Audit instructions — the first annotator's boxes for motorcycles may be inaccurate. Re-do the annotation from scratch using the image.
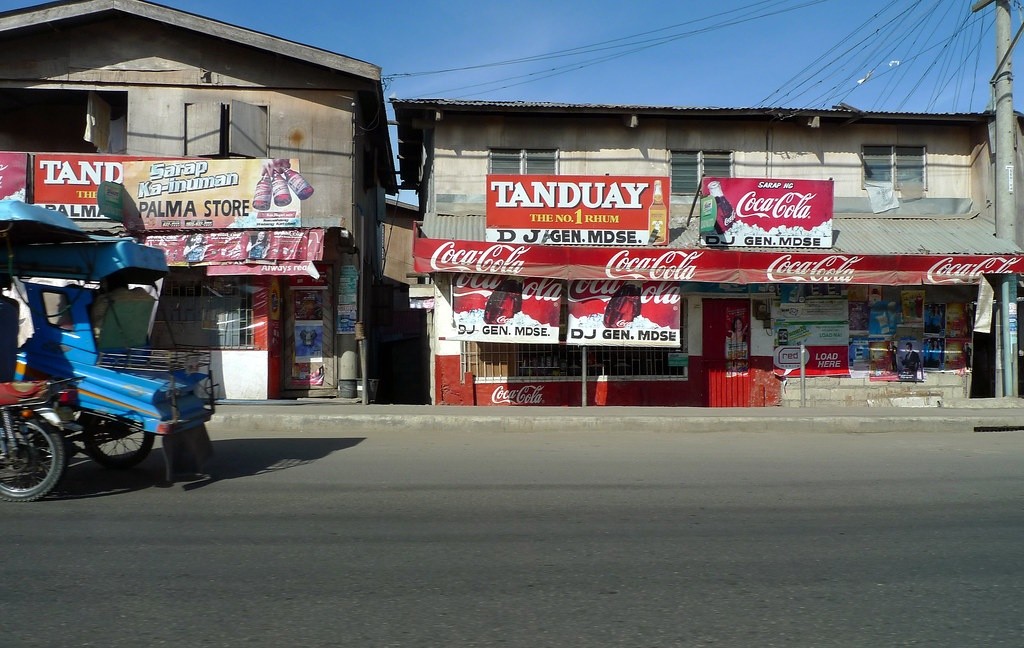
[0,376,87,504]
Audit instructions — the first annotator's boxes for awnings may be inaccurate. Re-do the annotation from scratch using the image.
[411,215,1023,285]
[87,225,359,278]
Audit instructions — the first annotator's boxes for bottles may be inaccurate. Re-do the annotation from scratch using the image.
[272,169,292,206]
[280,166,314,200]
[649,180,667,244]
[708,182,737,236]
[252,170,272,210]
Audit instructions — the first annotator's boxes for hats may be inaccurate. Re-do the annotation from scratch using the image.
[965,343,969,345]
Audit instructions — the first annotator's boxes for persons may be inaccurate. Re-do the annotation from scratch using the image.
[728,316,743,342]
[183,233,210,263]
[246,231,271,259]
[929,305,942,333]
[899,342,919,381]
[56,270,128,330]
[929,339,942,368]
